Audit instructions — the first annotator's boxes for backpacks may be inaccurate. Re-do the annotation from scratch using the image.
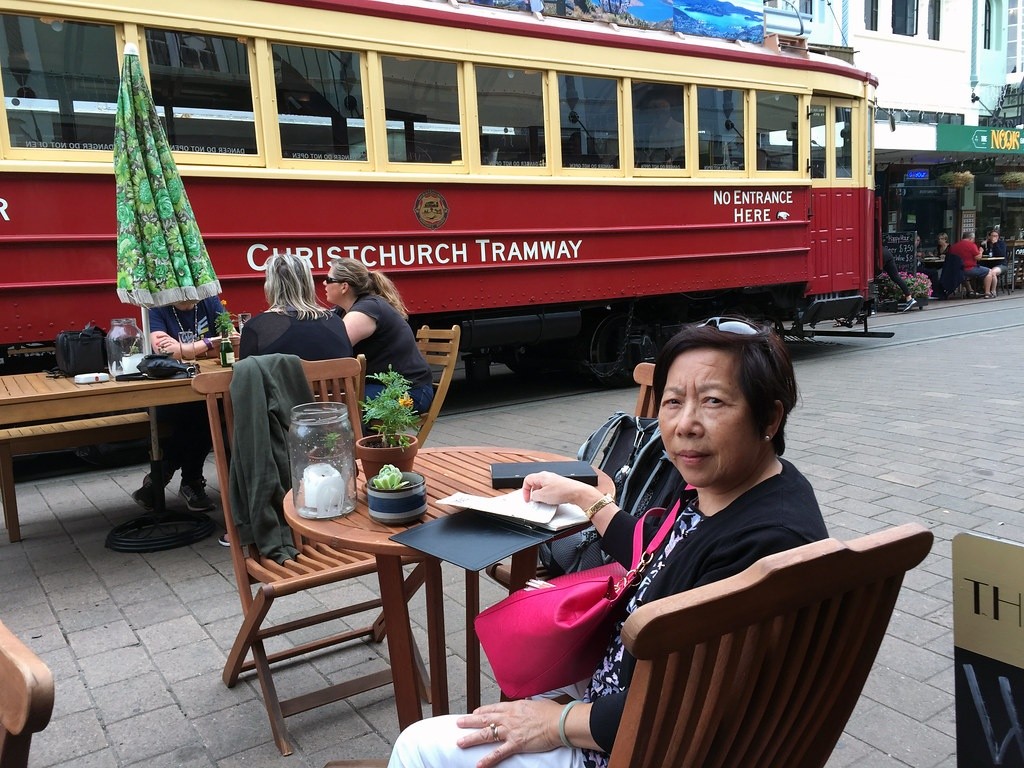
[538,410,689,578]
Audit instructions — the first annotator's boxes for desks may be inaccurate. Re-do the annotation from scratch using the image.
[0,353,240,426]
[283,445,615,717]
[924,257,1005,262]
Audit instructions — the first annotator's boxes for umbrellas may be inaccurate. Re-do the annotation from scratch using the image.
[114,43,223,362]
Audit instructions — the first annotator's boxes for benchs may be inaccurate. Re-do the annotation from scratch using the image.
[0,412,174,544]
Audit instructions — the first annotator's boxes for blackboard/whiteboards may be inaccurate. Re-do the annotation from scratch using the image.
[882,230,918,275]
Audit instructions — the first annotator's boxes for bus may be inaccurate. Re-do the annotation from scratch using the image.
[0,0,898,394]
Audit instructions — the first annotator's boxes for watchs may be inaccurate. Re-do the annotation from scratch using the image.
[585,492,616,519]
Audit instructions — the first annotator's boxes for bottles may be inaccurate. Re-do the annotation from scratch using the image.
[106,318,149,378]
[287,401,357,521]
[219,338,235,367]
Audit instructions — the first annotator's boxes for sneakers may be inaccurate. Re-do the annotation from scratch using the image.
[142,465,175,509]
[177,479,218,513]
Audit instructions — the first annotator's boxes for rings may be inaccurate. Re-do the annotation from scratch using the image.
[491,723,500,743]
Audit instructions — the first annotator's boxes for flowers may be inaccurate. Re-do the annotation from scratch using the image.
[359,364,423,449]
[941,171,975,190]
[214,300,234,338]
[877,272,933,296]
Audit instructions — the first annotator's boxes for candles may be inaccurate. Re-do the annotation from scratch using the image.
[120,353,145,374]
[303,462,344,509]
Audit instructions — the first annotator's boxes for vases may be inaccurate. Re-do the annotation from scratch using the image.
[210,336,240,365]
[355,433,418,496]
[951,176,964,188]
[914,294,929,307]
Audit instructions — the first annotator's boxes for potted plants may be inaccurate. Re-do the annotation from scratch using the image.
[367,464,428,524]
[1000,171,1024,190]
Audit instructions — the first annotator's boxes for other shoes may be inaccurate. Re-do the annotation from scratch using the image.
[903,298,917,313]
[967,290,998,299]
[217,534,231,548]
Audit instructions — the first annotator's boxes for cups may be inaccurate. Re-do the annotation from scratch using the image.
[238,313,251,335]
[178,332,196,364]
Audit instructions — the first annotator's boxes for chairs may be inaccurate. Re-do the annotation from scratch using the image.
[607,521,935,768]
[372,324,463,448]
[312,353,367,423]
[997,250,1012,295]
[191,357,432,756]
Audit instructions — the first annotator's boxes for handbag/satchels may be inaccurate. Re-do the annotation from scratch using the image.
[473,482,696,702]
[136,351,196,380]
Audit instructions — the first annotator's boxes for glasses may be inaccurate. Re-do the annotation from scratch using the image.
[989,234,999,237]
[938,237,945,241]
[680,314,762,334]
[324,275,343,285]
[917,239,921,243]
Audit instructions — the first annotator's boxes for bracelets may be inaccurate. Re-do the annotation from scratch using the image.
[559,700,584,749]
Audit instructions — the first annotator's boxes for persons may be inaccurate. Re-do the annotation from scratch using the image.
[915,229,1009,299]
[872,247,916,314]
[323,257,432,434]
[238,255,353,362]
[132,295,238,511]
[383,315,832,768]
